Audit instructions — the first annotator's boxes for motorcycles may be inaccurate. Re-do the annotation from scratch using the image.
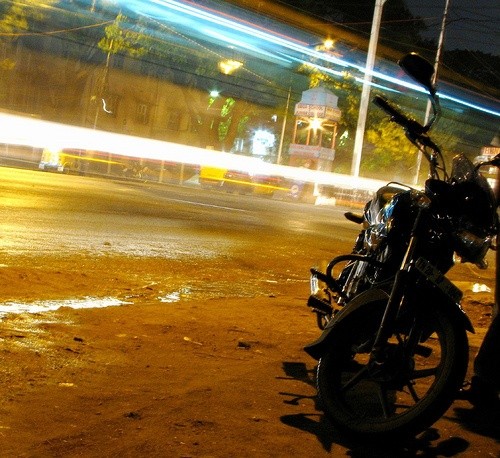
[306,51,500,447]
